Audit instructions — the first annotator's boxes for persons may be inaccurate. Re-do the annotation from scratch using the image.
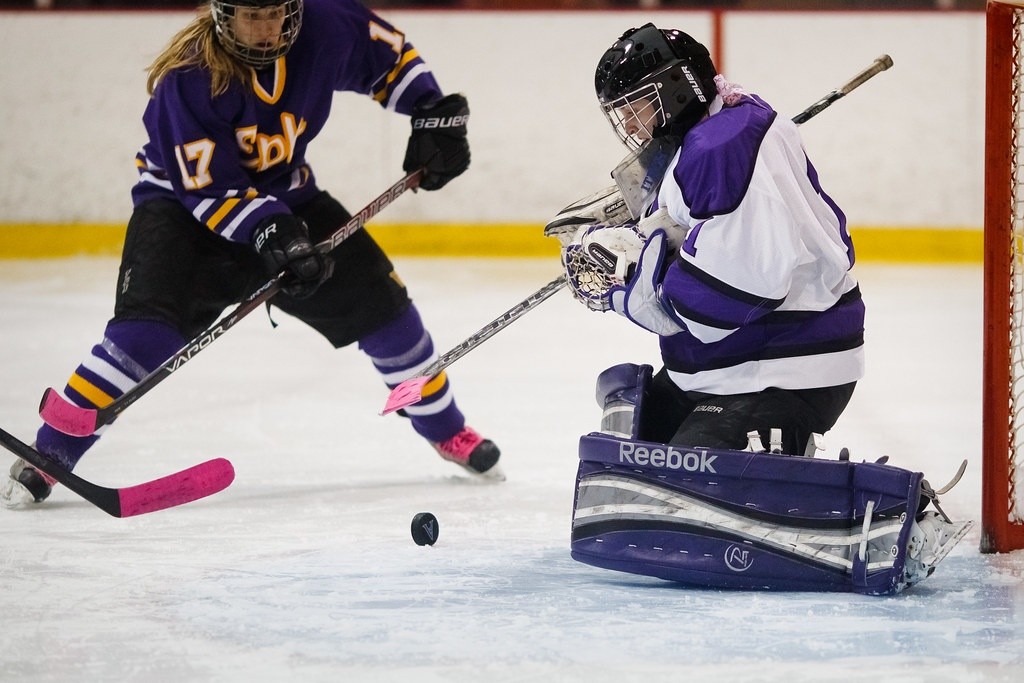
[560,23,864,455]
[0,0,500,509]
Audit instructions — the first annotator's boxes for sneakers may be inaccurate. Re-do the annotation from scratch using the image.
[431,425,506,484]
[0,442,61,510]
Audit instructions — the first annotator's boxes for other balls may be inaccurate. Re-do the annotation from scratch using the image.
[411,511,439,547]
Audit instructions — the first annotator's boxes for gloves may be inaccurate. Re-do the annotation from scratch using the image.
[248,213,335,302]
[403,94,471,192]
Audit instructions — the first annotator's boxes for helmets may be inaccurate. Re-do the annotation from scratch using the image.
[207,0,285,23]
[594,22,720,148]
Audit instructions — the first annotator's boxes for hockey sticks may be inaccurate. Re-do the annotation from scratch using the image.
[39,171,419,439]
[0,427,235,520]
[383,55,895,416]
